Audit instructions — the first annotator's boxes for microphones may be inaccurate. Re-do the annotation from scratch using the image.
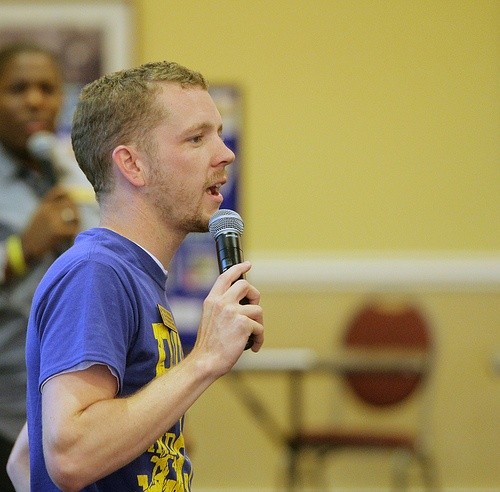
[27,132,61,189]
[208,209,254,351]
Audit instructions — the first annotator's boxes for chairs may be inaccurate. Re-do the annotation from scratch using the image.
[281,294,442,492]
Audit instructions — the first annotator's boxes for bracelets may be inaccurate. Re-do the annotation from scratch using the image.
[6,234,29,276]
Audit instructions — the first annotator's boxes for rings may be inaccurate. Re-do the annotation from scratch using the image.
[64,208,73,221]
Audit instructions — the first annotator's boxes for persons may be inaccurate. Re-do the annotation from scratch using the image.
[6,62,264,492]
[0,44,102,492]
